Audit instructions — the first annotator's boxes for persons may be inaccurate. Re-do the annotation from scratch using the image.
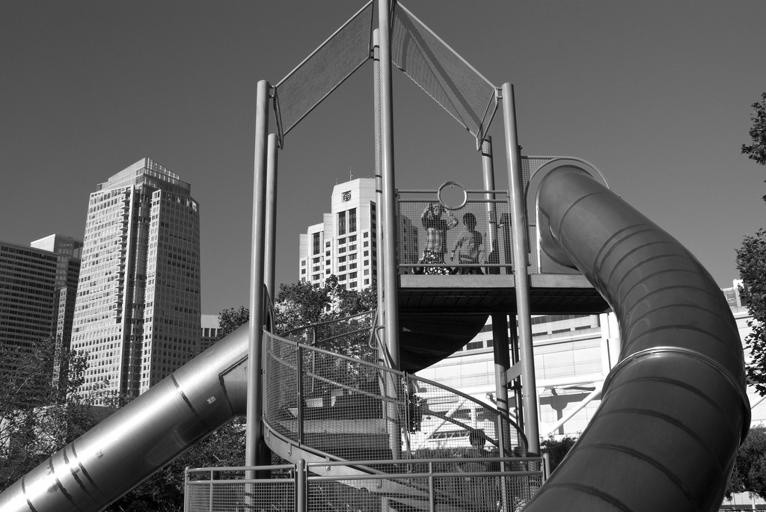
[421,202,459,275]
[451,213,485,275]
[455,429,493,482]
